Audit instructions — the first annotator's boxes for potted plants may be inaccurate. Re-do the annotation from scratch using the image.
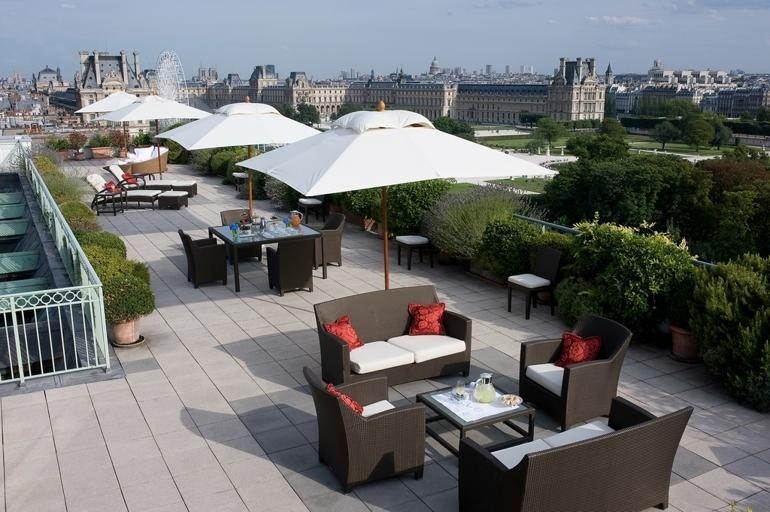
[48,132,127,161]
[666,277,700,359]
[31,156,154,348]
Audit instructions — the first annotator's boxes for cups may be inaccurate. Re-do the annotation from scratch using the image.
[455,381,465,398]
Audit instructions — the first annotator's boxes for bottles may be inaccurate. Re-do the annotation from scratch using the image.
[231,224,240,241]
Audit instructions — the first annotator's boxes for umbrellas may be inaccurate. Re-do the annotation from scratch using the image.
[73,88,142,157]
[91,94,218,182]
[234,96,565,291]
[153,99,325,221]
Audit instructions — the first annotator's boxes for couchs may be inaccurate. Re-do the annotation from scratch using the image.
[313,285,472,387]
[459,395,694,512]
[118,149,169,175]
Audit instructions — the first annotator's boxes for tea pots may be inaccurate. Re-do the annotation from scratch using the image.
[291,210,304,227]
[474,373,497,403]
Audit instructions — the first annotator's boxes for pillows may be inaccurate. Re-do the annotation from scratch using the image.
[408,302,447,336]
[118,146,165,165]
[323,315,364,351]
[556,332,601,368]
[326,384,363,416]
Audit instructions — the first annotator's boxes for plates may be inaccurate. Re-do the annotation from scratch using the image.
[499,395,524,407]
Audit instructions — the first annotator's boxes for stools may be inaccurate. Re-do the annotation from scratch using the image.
[172,181,197,198]
[158,191,189,210]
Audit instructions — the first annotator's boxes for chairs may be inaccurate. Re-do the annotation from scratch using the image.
[507,246,563,320]
[302,365,426,495]
[266,238,314,296]
[84,164,172,210]
[177,229,227,289]
[232,167,436,270]
[519,313,634,432]
[221,209,262,265]
